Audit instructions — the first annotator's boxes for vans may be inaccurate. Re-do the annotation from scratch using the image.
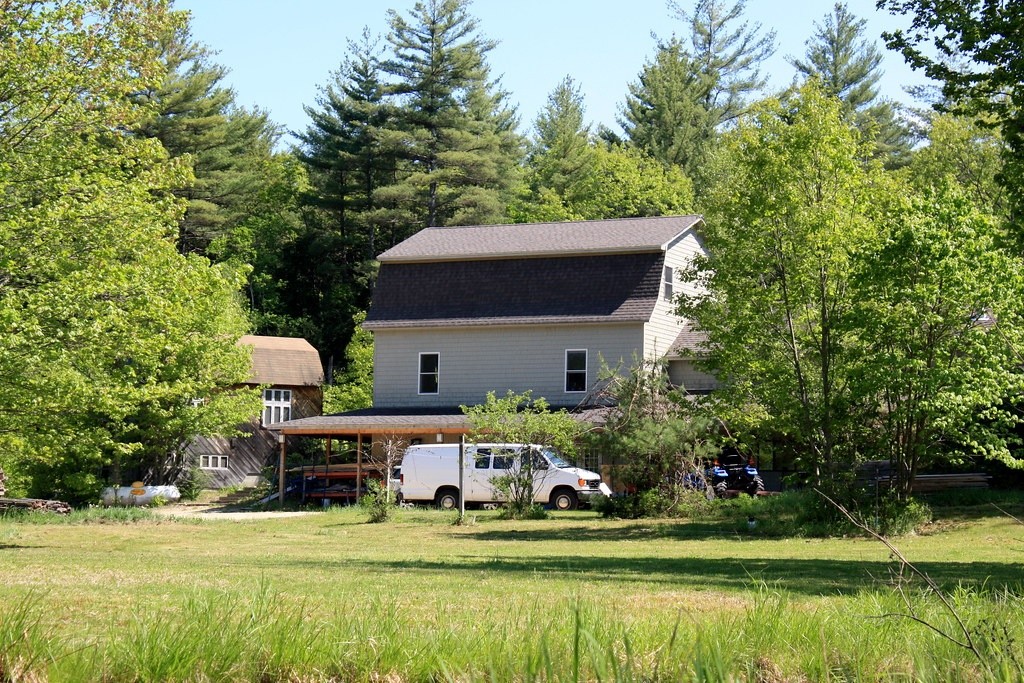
[399,444,602,509]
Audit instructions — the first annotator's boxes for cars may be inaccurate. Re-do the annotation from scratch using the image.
[389,466,402,504]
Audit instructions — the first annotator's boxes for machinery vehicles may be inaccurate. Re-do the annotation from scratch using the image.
[684,446,765,499]
